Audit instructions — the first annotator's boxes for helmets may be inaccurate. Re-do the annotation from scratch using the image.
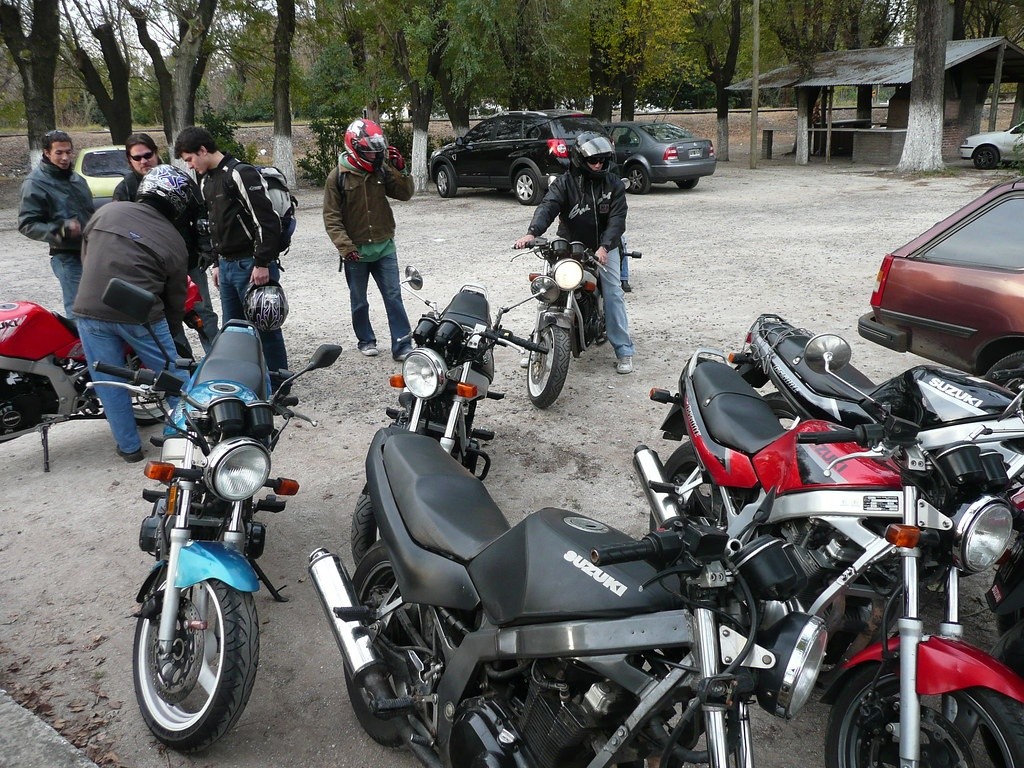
[345,118,385,174]
[570,131,610,177]
[136,163,204,220]
[244,278,289,331]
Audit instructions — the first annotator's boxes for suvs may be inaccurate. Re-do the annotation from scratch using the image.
[429,111,618,207]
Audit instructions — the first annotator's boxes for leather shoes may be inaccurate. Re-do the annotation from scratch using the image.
[621,281,631,292]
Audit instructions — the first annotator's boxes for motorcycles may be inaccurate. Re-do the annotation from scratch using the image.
[350,264,560,582]
[0,274,218,473]
[305,422,776,768]
[509,237,642,409]
[85,275,344,755]
[631,313,1024,768]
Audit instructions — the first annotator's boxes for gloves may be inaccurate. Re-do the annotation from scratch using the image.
[388,146,404,170]
[197,250,213,274]
[345,251,359,267]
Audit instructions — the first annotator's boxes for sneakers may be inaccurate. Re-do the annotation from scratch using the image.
[520,358,529,367]
[360,342,378,356]
[616,356,633,373]
[394,347,413,360]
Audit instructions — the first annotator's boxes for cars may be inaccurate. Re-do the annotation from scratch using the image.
[75,145,132,212]
[599,120,718,194]
[857,175,1024,395]
[957,122,1024,169]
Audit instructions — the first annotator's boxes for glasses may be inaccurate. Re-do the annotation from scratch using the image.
[584,157,605,165]
[45,130,63,136]
[129,151,154,161]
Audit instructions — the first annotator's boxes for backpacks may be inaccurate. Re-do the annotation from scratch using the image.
[225,160,297,253]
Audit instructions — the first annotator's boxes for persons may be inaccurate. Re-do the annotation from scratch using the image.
[18,130,97,321]
[174,126,292,395]
[72,164,202,463]
[323,118,414,364]
[514,129,633,375]
[112,133,220,365]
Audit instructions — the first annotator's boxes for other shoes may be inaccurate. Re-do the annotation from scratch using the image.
[117,444,144,463]
